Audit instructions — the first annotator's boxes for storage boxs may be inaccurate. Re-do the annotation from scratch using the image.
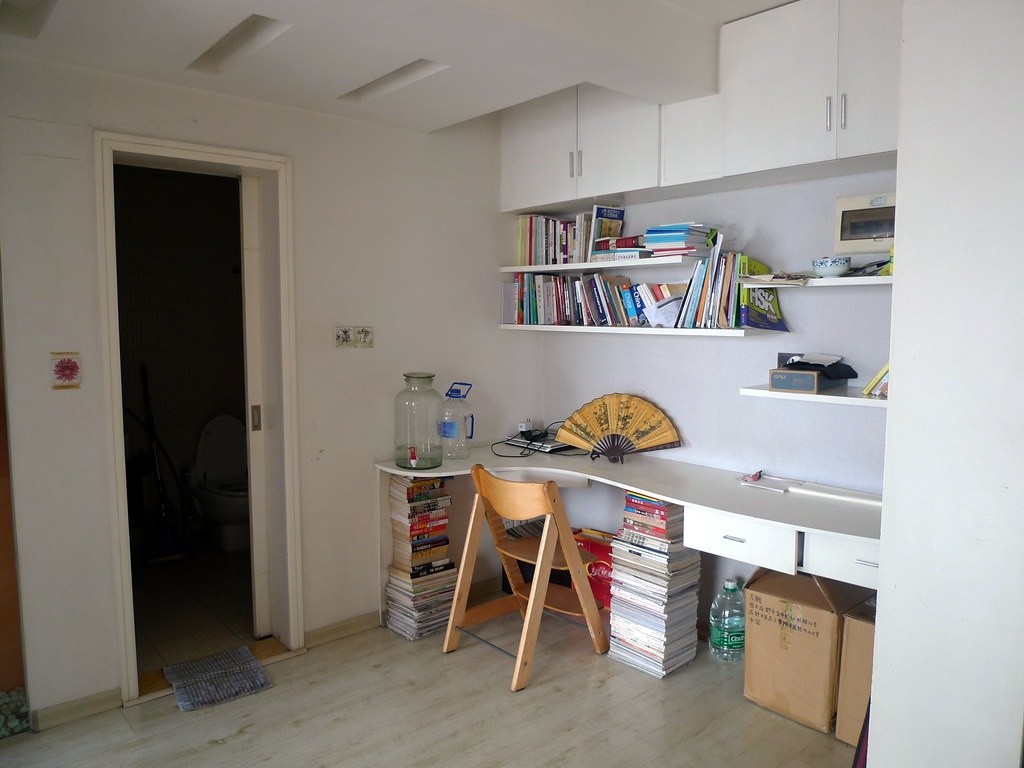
[572,528,613,607]
[501,518,581,595]
[835,596,876,748]
[742,566,876,733]
[769,369,848,395]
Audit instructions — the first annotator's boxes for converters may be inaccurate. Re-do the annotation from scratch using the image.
[525,430,547,440]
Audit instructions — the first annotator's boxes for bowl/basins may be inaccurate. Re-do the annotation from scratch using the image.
[812,257,851,278]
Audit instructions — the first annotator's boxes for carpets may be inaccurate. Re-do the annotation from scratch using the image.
[162,645,271,712]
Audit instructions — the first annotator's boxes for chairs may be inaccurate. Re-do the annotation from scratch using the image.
[443,463,609,691]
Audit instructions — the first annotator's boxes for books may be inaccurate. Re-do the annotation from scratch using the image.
[500,273,689,328]
[644,221,720,257]
[385,475,457,642]
[590,233,653,261]
[607,490,703,679]
[673,252,790,332]
[862,356,890,400]
[516,204,625,267]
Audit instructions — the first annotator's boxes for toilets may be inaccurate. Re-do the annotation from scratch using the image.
[191,415,248,553]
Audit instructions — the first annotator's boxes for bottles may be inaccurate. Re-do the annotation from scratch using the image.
[441,382,474,460]
[890,244,894,275]
[395,372,444,469]
[708,579,746,663]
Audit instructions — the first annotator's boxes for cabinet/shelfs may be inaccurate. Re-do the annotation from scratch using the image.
[498,0,898,406]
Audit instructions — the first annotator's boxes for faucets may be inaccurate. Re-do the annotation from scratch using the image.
[408,447,418,468]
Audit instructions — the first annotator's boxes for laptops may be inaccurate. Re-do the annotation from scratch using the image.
[505,429,578,453]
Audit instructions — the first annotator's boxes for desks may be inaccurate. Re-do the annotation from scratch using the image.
[374,444,882,627]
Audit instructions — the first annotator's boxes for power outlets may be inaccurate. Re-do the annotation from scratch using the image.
[504,519,527,529]
